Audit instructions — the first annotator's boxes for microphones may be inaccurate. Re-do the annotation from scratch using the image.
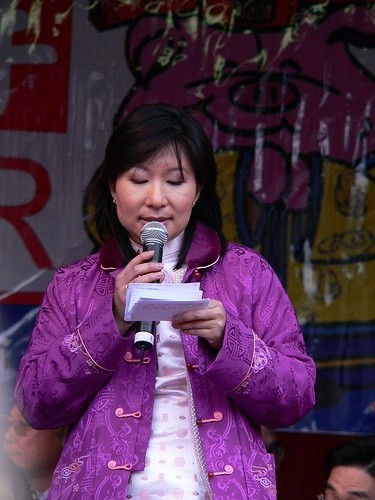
[132,220,170,366]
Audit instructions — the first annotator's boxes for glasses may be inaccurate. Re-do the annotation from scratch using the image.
[319,494,336,500]
[267,442,281,453]
[2,419,30,437]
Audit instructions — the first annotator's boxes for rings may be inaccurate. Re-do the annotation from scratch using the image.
[125,284,130,294]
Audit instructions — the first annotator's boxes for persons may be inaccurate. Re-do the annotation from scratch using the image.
[2,399,73,500]
[13,98,316,500]
[324,444,375,500]
